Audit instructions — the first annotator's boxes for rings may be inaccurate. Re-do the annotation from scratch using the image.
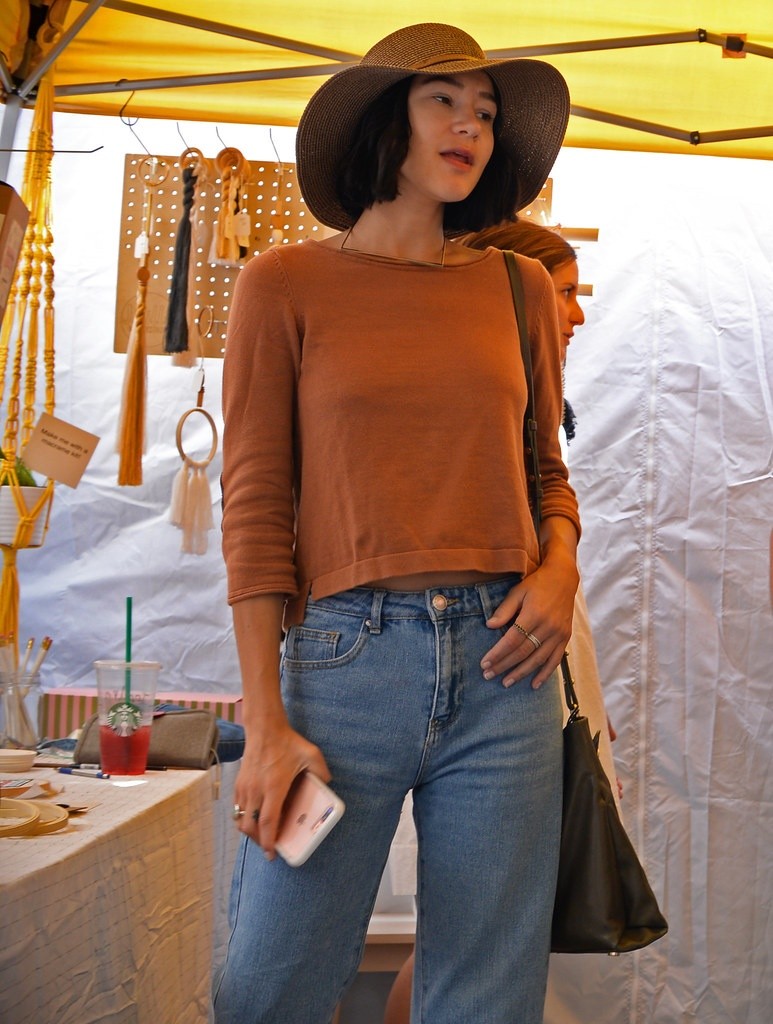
[232,803,244,819]
[527,634,541,647]
[513,623,528,636]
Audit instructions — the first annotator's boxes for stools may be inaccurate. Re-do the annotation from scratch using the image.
[333,913,417,1024]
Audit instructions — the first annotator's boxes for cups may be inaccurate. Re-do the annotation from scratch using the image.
[94,660,161,776]
[1,672,43,747]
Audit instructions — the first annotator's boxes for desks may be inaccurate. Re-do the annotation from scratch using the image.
[0,757,213,1024]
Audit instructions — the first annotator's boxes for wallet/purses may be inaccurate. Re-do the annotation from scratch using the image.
[75,707,221,771]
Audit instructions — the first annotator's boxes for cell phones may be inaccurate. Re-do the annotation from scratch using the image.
[268,767,346,872]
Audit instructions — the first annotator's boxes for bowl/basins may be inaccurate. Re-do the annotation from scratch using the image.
[0,749,38,772]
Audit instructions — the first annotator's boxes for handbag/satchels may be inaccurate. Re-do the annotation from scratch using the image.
[547,711,670,954]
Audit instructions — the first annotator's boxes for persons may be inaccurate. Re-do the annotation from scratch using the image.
[383,220,618,1024]
[208,23,582,1022]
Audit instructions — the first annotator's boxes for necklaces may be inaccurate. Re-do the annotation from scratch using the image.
[340,222,447,267]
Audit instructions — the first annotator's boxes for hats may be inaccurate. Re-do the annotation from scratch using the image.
[296,24,572,237]
